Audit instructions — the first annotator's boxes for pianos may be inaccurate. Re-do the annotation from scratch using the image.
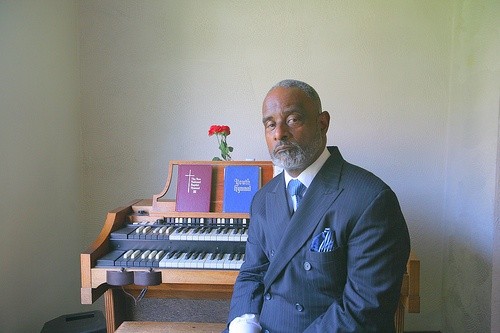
[78,160,420,333]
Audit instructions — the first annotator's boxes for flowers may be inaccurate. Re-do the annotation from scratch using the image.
[208,125,234,161]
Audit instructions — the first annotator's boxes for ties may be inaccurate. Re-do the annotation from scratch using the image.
[288,179,306,213]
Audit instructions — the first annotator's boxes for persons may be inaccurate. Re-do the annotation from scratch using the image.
[219,79,411,333]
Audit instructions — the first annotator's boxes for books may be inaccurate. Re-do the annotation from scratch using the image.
[222,165,262,214]
[175,164,213,212]
